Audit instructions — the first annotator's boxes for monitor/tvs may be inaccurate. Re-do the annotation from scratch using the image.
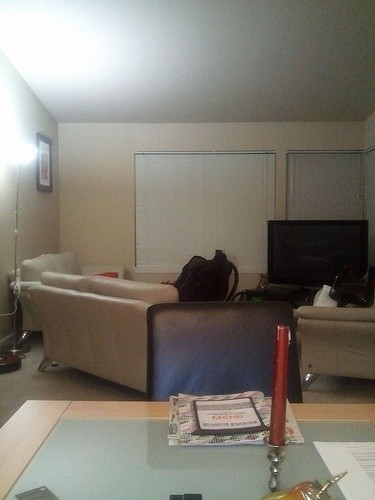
[267,219,368,288]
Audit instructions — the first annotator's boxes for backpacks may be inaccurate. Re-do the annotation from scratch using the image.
[174,249,239,302]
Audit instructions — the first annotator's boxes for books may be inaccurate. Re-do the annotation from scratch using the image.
[167,392,305,446]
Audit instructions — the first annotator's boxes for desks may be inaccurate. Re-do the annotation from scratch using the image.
[1,399,374,500]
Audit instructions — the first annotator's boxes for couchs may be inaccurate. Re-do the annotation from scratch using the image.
[9,251,126,350]
[30,270,180,391]
[294,306,375,388]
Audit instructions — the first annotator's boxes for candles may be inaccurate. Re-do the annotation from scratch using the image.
[270,325,289,445]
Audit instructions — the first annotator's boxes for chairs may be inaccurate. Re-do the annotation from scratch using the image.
[148,303,304,404]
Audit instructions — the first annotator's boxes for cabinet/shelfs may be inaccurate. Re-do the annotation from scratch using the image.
[246,288,375,305]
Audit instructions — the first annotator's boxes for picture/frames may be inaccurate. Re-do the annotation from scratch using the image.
[35,134,53,193]
